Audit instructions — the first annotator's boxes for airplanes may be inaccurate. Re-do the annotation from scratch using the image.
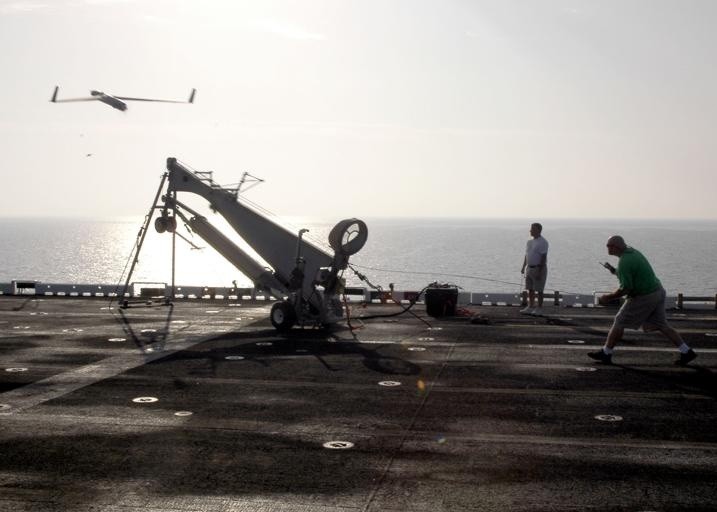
[45,82,199,114]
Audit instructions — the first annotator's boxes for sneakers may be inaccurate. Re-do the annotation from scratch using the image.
[676,348,699,365]
[587,348,613,362]
[531,307,545,314]
[520,306,534,314]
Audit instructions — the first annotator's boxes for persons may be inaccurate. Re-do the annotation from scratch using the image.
[519,222,550,317]
[588,235,699,367]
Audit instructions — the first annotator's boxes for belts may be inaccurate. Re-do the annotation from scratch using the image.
[528,264,541,268]
